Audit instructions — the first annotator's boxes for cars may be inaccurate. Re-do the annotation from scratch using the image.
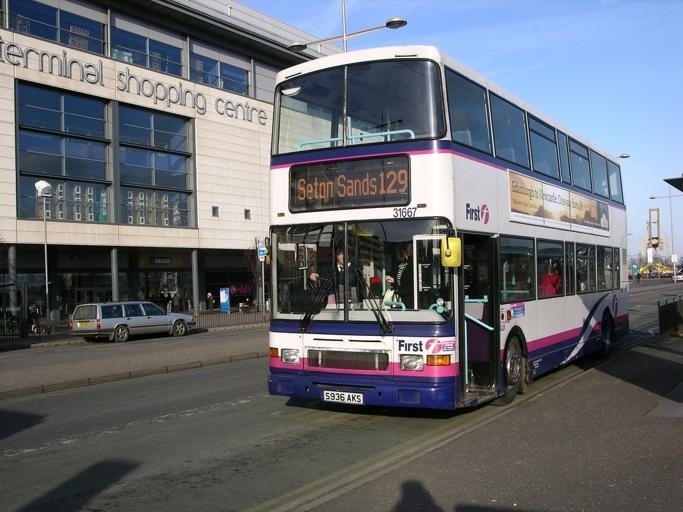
[67,301,197,344]
[672,270,683,284]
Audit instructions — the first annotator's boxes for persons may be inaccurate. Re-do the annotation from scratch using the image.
[543,259,562,296]
[310,246,357,286]
[385,242,414,309]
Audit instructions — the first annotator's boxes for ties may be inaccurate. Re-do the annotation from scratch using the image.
[339,265,343,275]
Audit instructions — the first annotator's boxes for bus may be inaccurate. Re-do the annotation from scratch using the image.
[269,44,628,412]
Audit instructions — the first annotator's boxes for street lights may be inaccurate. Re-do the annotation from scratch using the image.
[283,1,407,53]
[649,186,675,255]
[617,151,630,161]
[35,178,53,336]
